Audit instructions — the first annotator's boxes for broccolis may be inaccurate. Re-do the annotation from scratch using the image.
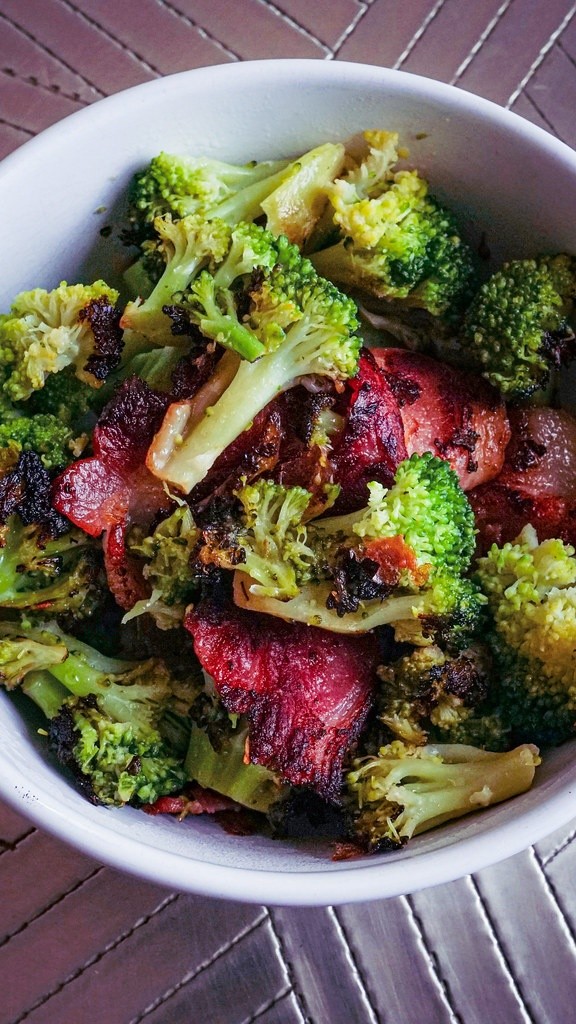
[0,133,576,849]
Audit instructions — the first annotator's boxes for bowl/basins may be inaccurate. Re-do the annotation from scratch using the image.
[0,58,575,905]
